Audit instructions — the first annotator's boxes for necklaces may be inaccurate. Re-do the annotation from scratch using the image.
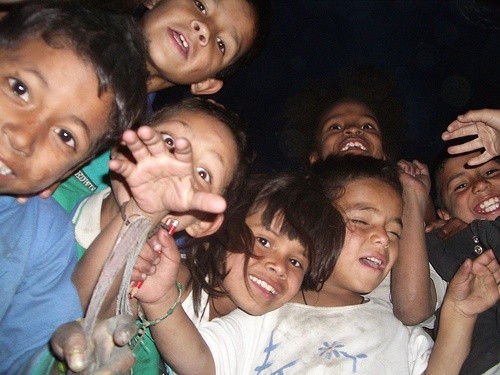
[299,284,365,305]
[212,296,224,316]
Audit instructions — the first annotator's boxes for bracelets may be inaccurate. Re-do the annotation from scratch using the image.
[134,278,182,329]
[121,200,131,227]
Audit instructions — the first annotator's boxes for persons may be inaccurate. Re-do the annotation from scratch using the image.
[296,99,404,171]
[89,99,251,245]
[427,136,500,244]
[283,154,411,308]
[208,171,325,317]
[1,6,140,200]
[144,0,265,103]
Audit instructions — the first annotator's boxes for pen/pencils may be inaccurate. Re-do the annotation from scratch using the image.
[126,218,179,300]
